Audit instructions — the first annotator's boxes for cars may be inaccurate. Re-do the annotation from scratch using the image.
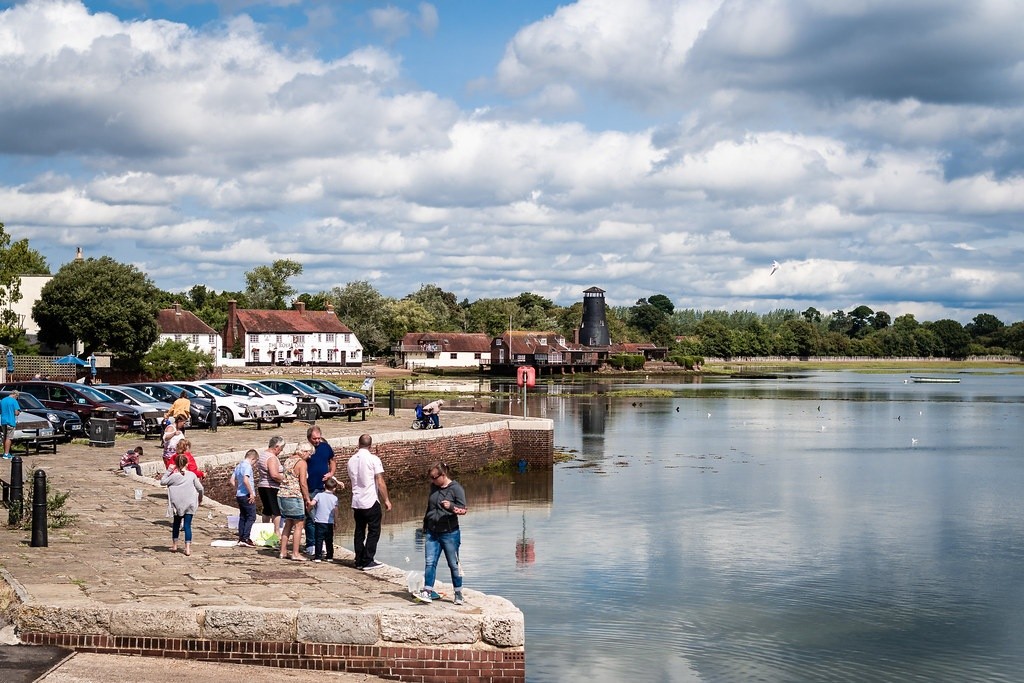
[0,400,55,446]
[118,379,370,428]
[366,357,377,361]
[90,386,191,435]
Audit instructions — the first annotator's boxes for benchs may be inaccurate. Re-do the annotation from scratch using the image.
[144,411,186,440]
[248,405,291,431]
[1,422,67,457]
[340,398,373,420]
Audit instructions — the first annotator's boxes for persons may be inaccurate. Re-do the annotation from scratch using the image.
[301,425,336,555]
[412,461,467,606]
[257,436,286,541]
[32,374,51,381]
[346,434,392,571]
[167,439,206,531]
[422,399,444,428]
[84,374,95,386]
[160,454,204,557]
[230,449,259,548]
[308,479,339,563]
[120,446,143,476]
[0,390,21,459]
[161,391,191,448]
[162,414,187,473]
[277,442,315,561]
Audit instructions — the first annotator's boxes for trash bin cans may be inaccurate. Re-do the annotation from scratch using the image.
[89,407,118,448]
[296,394,316,426]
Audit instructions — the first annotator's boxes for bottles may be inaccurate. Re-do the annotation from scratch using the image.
[208,510,213,519]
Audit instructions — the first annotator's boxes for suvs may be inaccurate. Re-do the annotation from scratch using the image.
[0,391,83,443]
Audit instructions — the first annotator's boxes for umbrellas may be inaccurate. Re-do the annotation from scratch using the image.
[91,353,97,383]
[53,353,90,383]
[7,349,14,383]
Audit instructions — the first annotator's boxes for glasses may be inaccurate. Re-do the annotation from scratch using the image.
[430,472,442,479]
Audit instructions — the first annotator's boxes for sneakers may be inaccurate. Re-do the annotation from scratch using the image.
[454,591,462,604]
[411,590,432,603]
[238,539,257,547]
[362,561,384,570]
[3,453,15,459]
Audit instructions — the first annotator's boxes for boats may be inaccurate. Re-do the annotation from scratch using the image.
[910,376,961,383]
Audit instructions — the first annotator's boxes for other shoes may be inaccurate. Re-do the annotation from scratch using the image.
[306,544,327,556]
[323,557,333,561]
[312,559,321,562]
[292,555,307,560]
[280,552,292,558]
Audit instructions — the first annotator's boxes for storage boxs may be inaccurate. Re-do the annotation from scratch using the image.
[226,515,240,528]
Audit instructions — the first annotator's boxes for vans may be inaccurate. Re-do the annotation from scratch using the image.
[0,381,143,439]
